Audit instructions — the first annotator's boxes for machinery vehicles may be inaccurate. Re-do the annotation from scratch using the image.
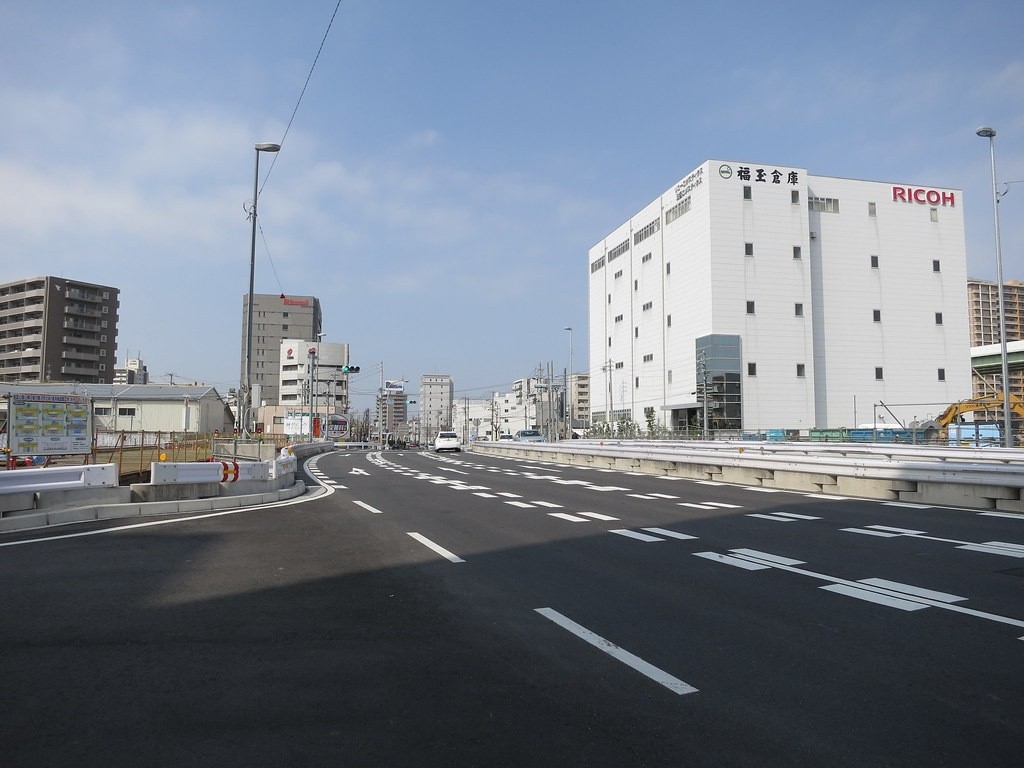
[922,390,1024,448]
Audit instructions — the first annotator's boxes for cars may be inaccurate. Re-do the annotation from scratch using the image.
[433,431,462,453]
[499,434,514,442]
[513,430,547,443]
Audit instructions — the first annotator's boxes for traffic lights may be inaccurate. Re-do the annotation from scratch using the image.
[341,365,361,373]
[406,400,416,404]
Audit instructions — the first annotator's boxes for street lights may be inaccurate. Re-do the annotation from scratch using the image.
[975,125,1012,448]
[563,327,572,439]
[242,142,283,441]
[314,332,327,418]
[385,379,410,428]
